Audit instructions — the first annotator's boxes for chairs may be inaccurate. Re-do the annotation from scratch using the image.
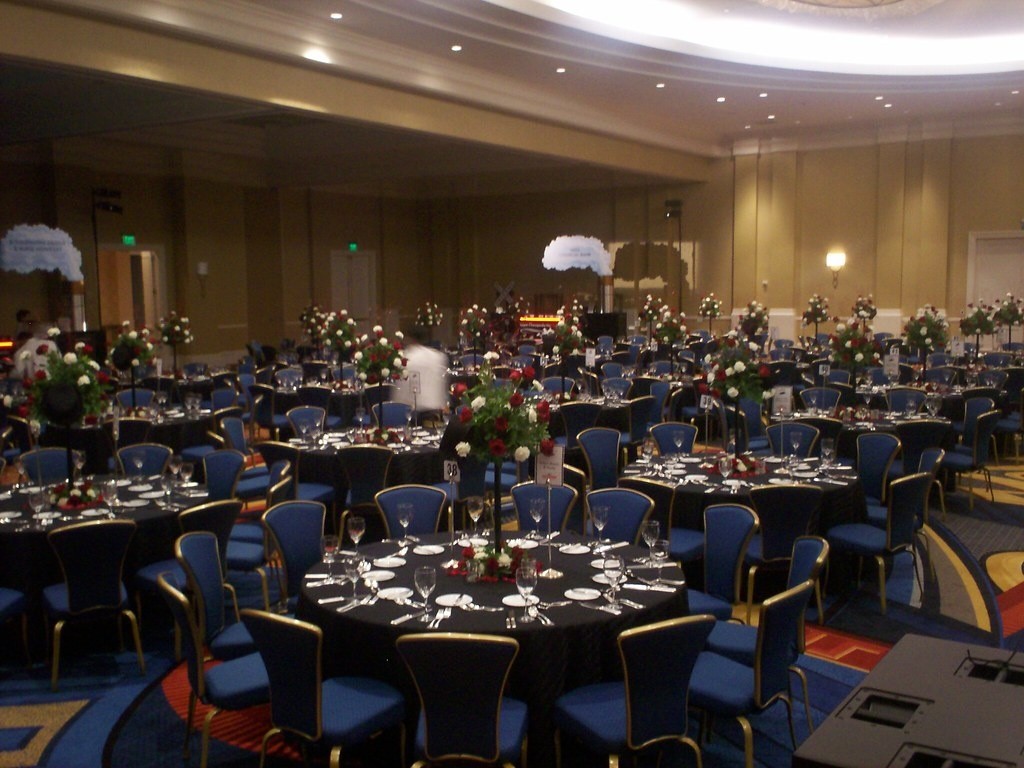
[0,331,1024,768]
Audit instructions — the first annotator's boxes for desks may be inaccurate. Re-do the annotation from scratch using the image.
[633,370,696,405]
[534,392,634,437]
[82,404,216,458]
[286,424,451,534]
[770,406,957,494]
[298,530,691,698]
[620,450,868,612]
[141,367,234,405]
[854,382,957,411]
[275,377,378,424]
[0,473,215,647]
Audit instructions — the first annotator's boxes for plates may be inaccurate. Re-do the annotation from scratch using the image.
[506,539,539,549]
[0,327,1024,528]
[413,544,445,555]
[361,571,395,581]
[501,594,540,606]
[458,538,489,548]
[590,559,620,569]
[377,587,413,600]
[592,573,627,584]
[372,557,406,567]
[559,545,591,554]
[564,587,601,600]
[435,593,473,606]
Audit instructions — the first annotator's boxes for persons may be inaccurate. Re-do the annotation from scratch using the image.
[16,310,31,332]
[14,332,61,380]
[395,325,445,410]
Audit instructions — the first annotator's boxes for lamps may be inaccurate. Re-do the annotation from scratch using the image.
[664,200,682,219]
[825,242,846,290]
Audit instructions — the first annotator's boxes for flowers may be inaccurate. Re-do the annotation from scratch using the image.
[851,293,878,320]
[415,301,444,327]
[364,425,405,445]
[828,405,886,423]
[155,312,193,345]
[104,320,163,374]
[637,292,670,322]
[697,292,770,478]
[121,405,151,417]
[827,318,882,372]
[299,301,409,384]
[656,306,690,344]
[330,379,351,390]
[4,328,116,437]
[163,368,184,378]
[551,295,592,359]
[457,305,494,338]
[449,351,556,462]
[800,294,831,325]
[44,479,104,510]
[447,542,542,583]
[898,292,1024,394]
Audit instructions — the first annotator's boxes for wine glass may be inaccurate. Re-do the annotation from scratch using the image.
[414,566,436,622]
[588,506,609,550]
[526,496,546,540]
[604,554,625,610]
[346,517,365,561]
[320,534,340,584]
[640,521,660,568]
[395,502,413,546]
[343,558,364,606]
[467,496,484,539]
[516,566,538,624]
[650,539,669,593]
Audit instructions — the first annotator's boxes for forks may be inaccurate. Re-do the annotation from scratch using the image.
[303,529,687,629]
